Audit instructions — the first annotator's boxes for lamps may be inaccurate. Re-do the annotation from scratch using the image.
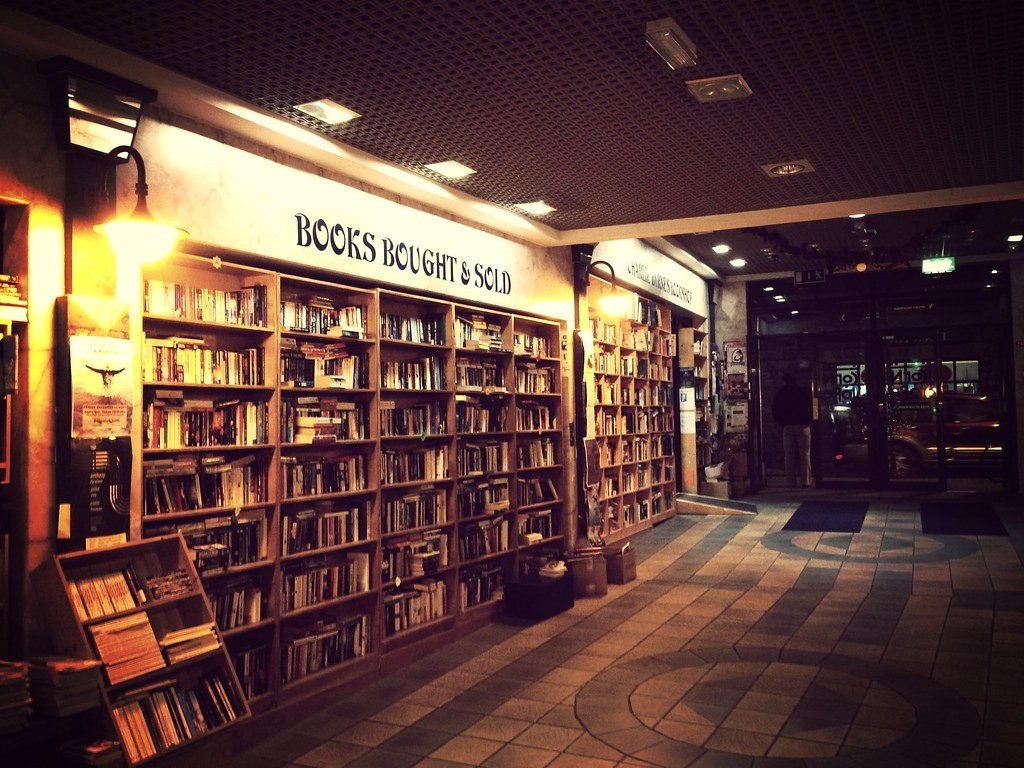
[643,17,699,70]
[91,194,191,267]
[760,158,815,179]
[33,56,158,165]
[684,73,754,103]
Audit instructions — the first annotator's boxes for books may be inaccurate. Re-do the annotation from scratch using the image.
[0,270,683,767]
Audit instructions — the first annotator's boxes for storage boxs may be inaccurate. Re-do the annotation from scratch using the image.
[504,546,636,619]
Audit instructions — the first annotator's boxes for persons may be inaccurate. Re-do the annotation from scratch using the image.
[771,372,813,488]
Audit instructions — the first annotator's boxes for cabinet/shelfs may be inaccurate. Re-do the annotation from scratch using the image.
[32,533,252,765]
[374,288,460,656]
[275,274,379,699]
[128,251,278,716]
[585,273,676,547]
[510,315,573,588]
[677,326,710,496]
[452,303,516,619]
